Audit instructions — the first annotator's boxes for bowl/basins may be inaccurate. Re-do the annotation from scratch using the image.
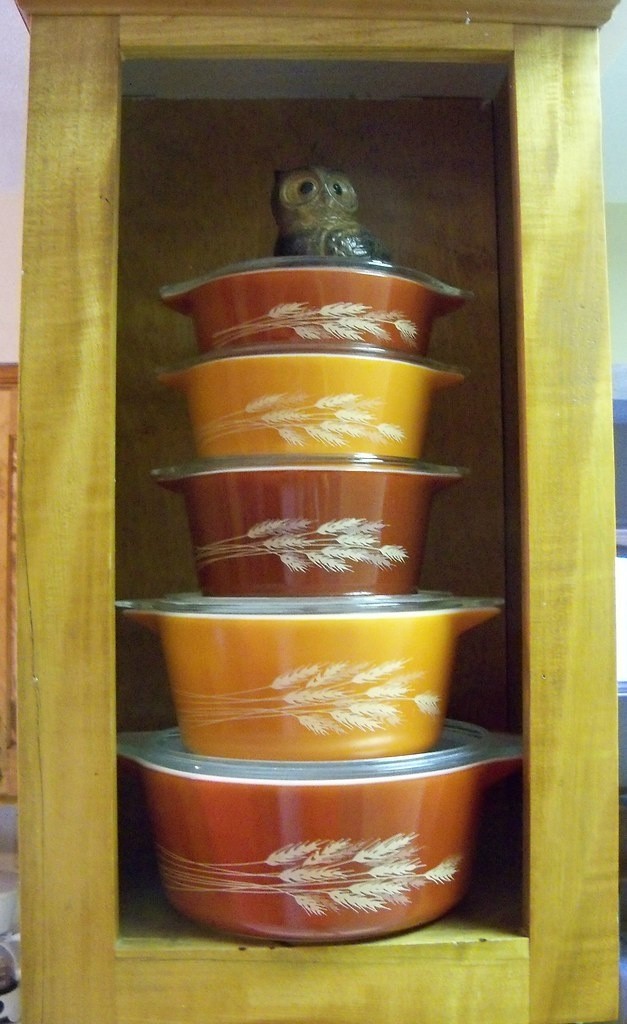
[117,257,527,942]
